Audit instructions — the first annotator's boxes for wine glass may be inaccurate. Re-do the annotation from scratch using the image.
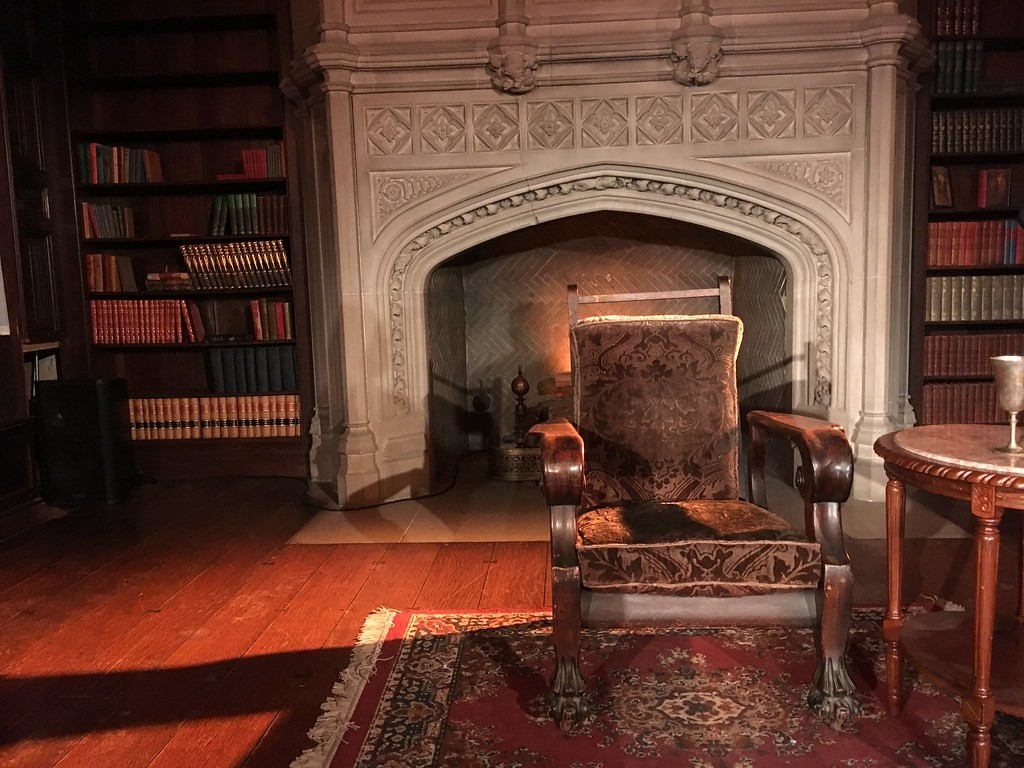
[989,354,1024,453]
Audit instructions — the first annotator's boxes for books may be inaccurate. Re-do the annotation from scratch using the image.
[920,0,1024,426]
[75,141,300,440]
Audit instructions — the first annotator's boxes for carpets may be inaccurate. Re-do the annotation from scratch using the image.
[284,593,1024,768]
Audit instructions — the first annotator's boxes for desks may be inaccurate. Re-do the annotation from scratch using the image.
[486,443,547,482]
[875,424,1024,768]
[19,339,58,398]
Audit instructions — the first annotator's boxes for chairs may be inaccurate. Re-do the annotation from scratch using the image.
[0,336,46,518]
[523,273,866,737]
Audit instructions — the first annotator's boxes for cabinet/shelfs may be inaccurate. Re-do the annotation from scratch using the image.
[49,0,317,483]
[909,0,1024,429]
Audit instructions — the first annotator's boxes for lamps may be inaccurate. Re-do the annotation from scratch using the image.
[512,364,530,448]
